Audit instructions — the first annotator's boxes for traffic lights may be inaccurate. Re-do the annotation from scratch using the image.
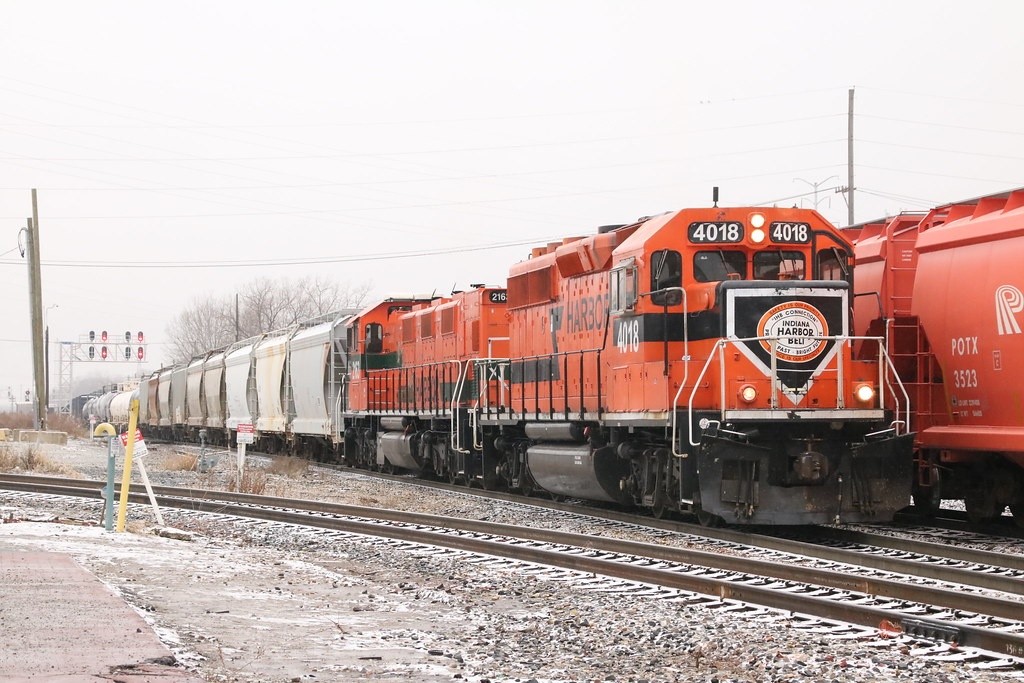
[138,347,144,359]
[138,331,143,342]
[102,346,108,359]
[102,331,107,342]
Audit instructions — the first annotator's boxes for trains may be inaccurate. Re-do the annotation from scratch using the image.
[80,187,921,529]
[785,184,1024,536]
